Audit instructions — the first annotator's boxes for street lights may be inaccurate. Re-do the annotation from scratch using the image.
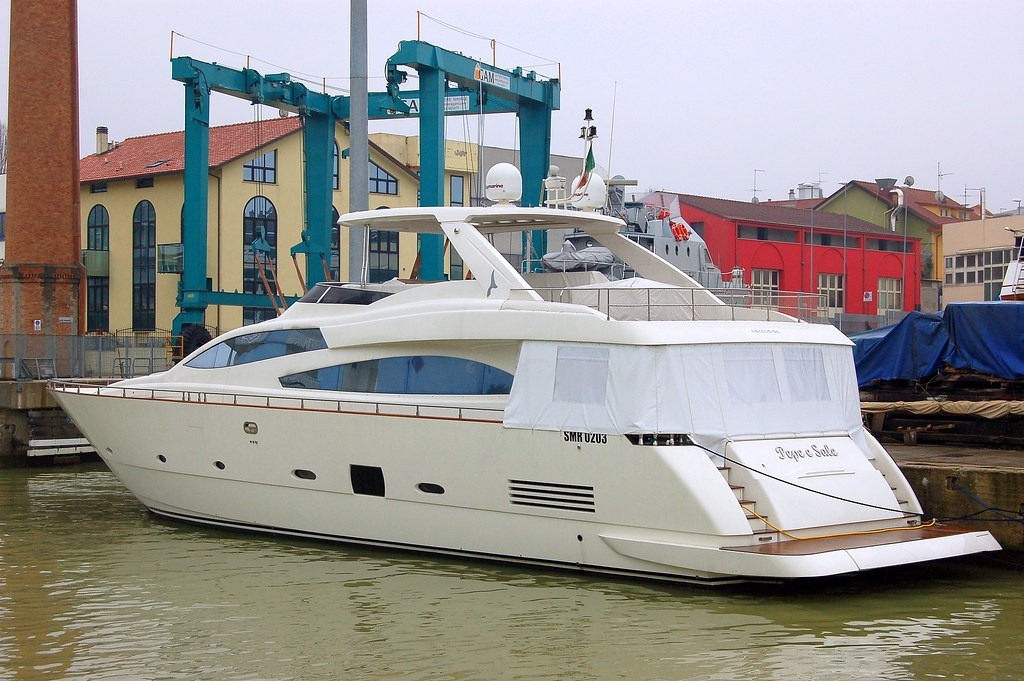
[802,181,814,324]
[899,185,909,312]
[837,181,849,321]
[1011,199,1022,215]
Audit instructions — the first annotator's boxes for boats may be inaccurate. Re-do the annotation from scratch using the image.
[44,79,1006,589]
[998,226,1024,301]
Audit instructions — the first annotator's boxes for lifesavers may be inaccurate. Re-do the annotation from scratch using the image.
[678,223,689,240]
[660,211,670,220]
[672,224,682,241]
